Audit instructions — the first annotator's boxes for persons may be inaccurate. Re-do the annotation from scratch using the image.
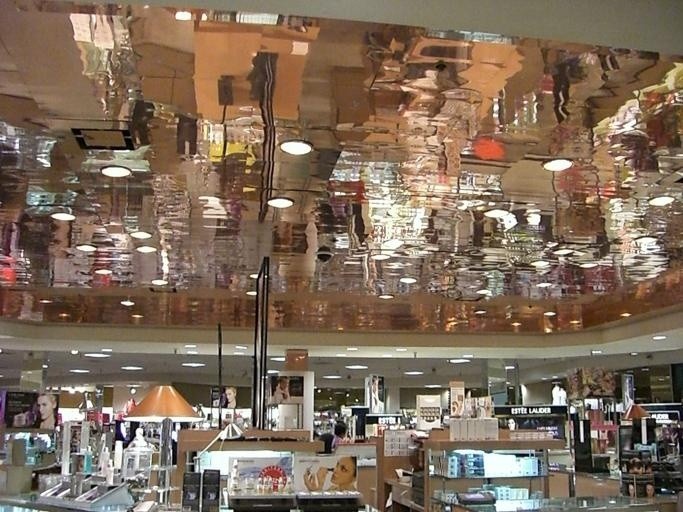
[629,483,636,504]
[371,375,378,410]
[331,421,353,448]
[223,387,237,409]
[273,376,289,404]
[593,373,616,397]
[304,458,356,492]
[34,394,58,429]
[644,482,654,505]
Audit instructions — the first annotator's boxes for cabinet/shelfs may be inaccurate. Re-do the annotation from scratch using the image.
[412,439,564,512]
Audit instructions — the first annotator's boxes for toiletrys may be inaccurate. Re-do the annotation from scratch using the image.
[49,432,159,505]
[227,461,292,497]
[467,469,484,475]
[13,413,26,428]
[466,456,483,463]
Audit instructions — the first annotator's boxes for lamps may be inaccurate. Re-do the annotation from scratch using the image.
[122,385,203,503]
[621,405,651,420]
[195,423,242,471]
